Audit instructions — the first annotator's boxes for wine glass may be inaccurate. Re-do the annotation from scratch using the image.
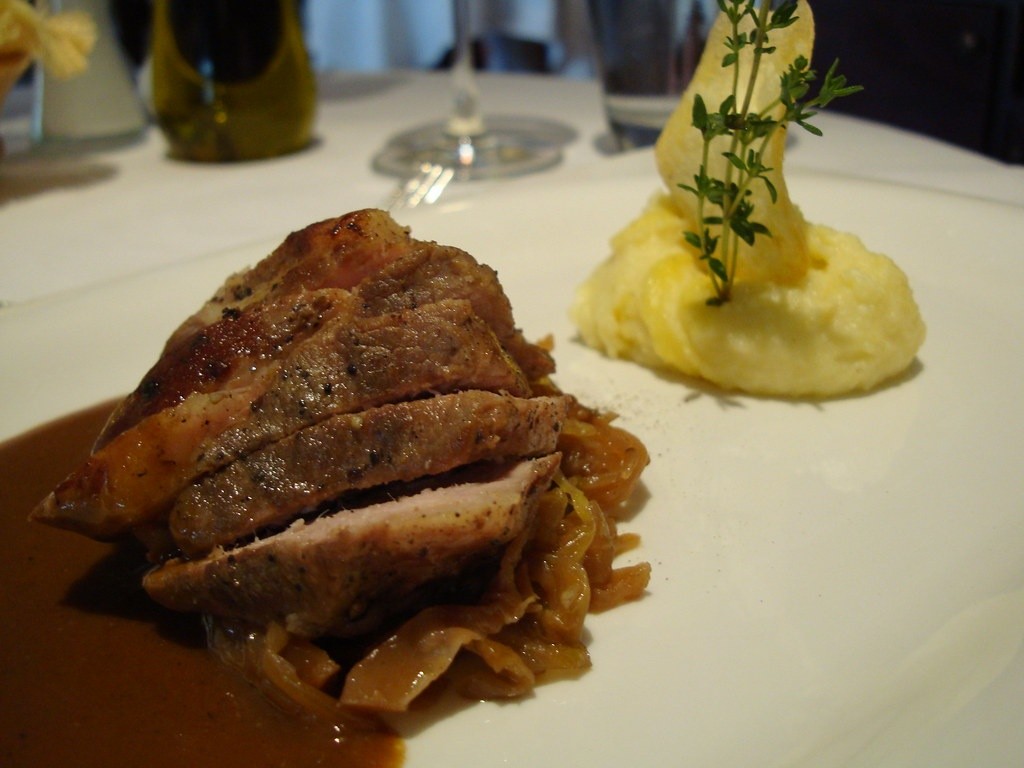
[366,0,569,180]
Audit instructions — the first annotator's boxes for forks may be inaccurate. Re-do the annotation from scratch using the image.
[382,163,456,215]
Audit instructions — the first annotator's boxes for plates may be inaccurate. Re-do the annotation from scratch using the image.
[0,168,1024,768]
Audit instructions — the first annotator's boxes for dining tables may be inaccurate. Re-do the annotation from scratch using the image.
[1,70,1024,767]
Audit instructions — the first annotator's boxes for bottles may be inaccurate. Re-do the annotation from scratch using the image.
[31,0,143,147]
[149,1,317,161]
[585,0,717,152]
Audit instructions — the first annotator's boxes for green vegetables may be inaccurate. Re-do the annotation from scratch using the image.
[676,0,865,305]
